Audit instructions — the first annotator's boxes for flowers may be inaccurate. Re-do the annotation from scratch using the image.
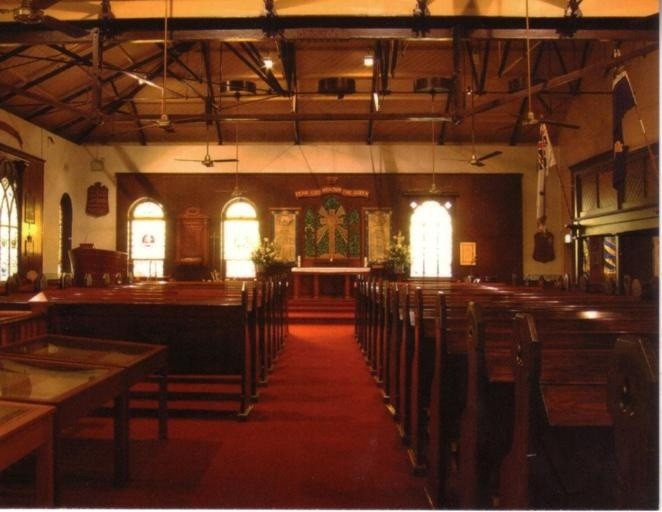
[379,228,412,266]
[250,235,284,268]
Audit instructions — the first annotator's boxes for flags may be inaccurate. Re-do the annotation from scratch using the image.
[535,116,556,233]
[611,48,635,210]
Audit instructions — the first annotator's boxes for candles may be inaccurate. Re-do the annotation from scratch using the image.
[363,256,367,267]
[298,255,301,268]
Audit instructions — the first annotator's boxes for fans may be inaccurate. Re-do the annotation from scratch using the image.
[174,126,240,168]
[120,2,202,132]
[497,0,580,134]
[439,75,503,168]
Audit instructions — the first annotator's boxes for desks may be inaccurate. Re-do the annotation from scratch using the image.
[0,400,60,509]
[291,267,372,301]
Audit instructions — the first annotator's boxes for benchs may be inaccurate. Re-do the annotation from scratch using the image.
[0,273,290,421]
[353,269,658,508]
